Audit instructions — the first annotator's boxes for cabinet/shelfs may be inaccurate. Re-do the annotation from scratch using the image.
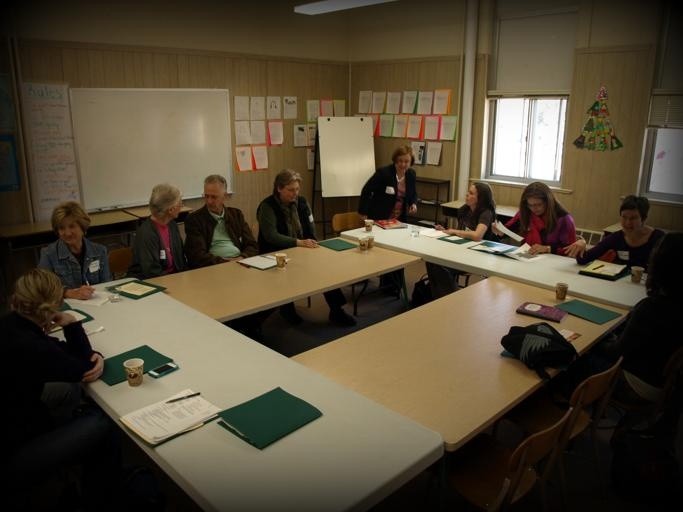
[406,176,451,228]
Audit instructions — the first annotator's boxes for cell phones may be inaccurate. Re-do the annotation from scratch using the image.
[148,362,178,378]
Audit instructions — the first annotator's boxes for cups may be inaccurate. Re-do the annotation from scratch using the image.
[630,266,644,284]
[122,357,144,387]
[275,252,286,268]
[359,234,374,251]
[554,282,568,300]
[363,219,374,231]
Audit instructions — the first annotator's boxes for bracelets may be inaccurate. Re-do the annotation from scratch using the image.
[547,245,551,253]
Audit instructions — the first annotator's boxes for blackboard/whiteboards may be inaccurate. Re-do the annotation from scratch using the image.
[68,87,235,214]
[317,116,376,199]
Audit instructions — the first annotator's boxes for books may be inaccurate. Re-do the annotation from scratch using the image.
[374,218,408,229]
[516,301,567,323]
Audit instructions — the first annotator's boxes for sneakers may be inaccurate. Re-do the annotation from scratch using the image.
[328,309,356,327]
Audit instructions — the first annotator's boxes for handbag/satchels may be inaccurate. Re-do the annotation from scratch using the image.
[500,322,575,370]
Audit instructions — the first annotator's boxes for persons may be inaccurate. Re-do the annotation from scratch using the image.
[256,167,356,327]
[564,195,667,274]
[37,203,110,300]
[0,268,104,450]
[560,233,681,409]
[358,146,418,290]
[127,184,183,279]
[184,175,280,346]
[491,181,576,256]
[426,182,495,298]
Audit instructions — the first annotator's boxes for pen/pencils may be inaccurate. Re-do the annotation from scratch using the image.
[85,280,90,286]
[495,219,497,236]
[166,392,200,404]
[593,264,605,270]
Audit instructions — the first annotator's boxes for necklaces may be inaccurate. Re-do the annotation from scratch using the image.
[395,173,405,185]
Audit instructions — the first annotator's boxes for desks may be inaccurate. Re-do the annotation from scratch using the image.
[1,203,194,255]
[35,212,651,512]
